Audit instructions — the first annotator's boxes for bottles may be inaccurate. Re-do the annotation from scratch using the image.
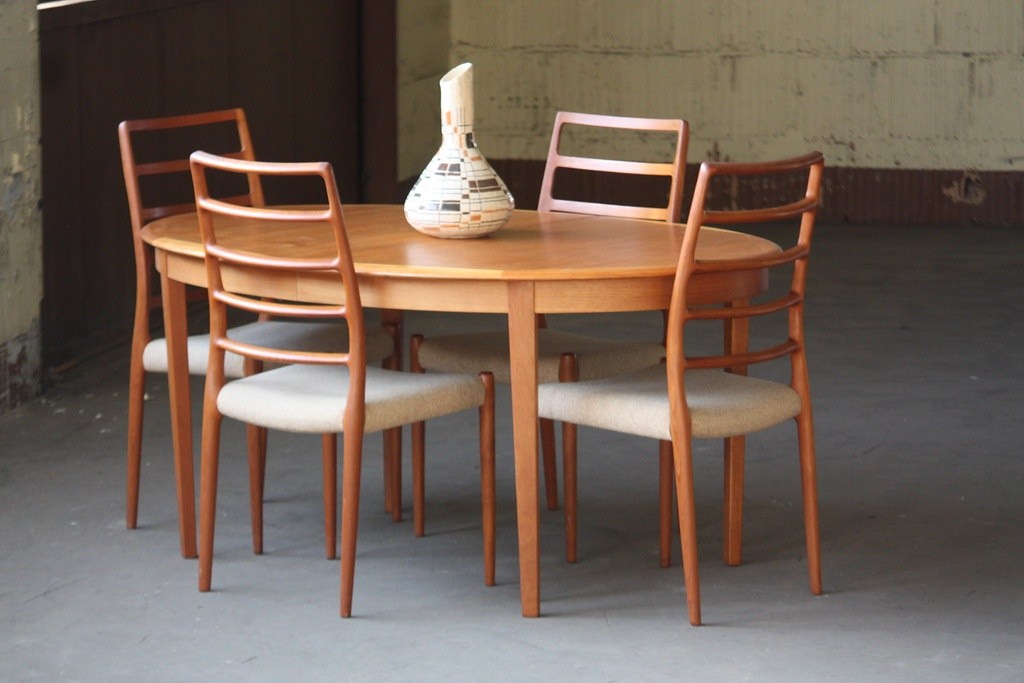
[404,64,515,238]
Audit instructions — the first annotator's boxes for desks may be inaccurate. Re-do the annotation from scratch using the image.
[135,204,787,617]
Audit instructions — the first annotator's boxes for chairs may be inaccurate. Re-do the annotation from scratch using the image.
[408,109,696,571]
[185,152,498,617]
[118,108,395,560]
[536,149,827,626]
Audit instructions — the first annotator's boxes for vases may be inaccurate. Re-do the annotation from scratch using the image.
[405,62,515,243]
